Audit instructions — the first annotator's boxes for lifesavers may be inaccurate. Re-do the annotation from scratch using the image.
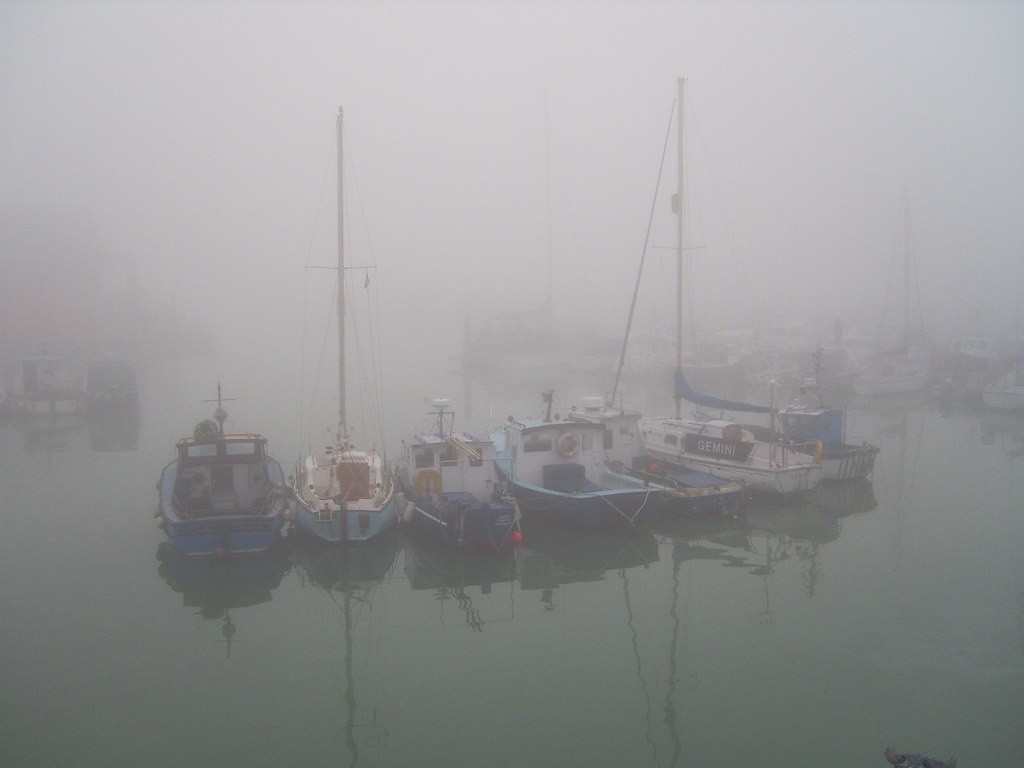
[557,432,581,458]
[413,467,442,494]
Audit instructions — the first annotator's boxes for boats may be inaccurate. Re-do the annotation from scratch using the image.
[288,73,1024,547]
[149,381,289,557]
